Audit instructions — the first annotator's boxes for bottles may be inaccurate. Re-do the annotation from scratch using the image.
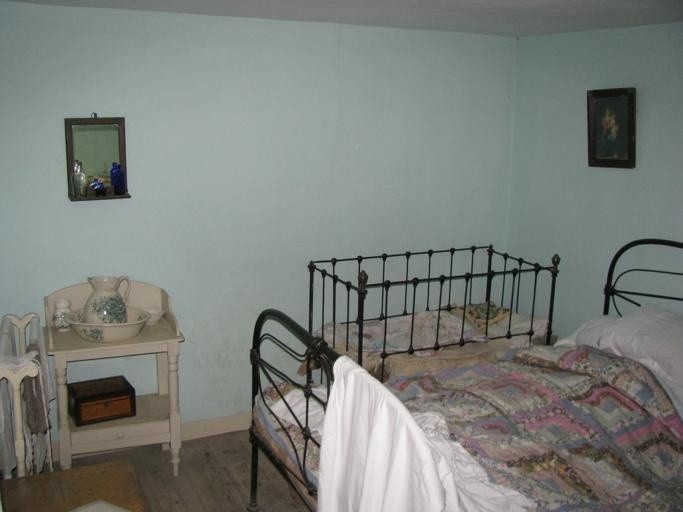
[94,182,106,197]
[112,162,125,196]
[72,162,87,197]
[53,299,73,333]
[110,162,118,185]
[89,178,99,190]
[72,159,82,173]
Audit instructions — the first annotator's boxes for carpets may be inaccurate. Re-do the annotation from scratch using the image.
[5,457,151,510]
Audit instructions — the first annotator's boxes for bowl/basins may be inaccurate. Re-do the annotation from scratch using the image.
[63,306,151,343]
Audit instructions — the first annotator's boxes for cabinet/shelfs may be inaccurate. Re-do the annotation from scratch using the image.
[43,278,187,479]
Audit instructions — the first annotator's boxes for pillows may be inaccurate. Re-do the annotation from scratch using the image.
[561,303,683,402]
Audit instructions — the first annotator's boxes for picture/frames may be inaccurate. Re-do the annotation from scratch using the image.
[585,86,636,169]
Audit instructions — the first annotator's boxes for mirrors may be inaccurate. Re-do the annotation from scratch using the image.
[66,116,128,202]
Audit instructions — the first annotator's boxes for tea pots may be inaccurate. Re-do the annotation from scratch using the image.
[81,275,132,324]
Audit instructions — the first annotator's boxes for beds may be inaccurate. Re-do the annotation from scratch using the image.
[250,238,683,510]
[301,243,564,355]
